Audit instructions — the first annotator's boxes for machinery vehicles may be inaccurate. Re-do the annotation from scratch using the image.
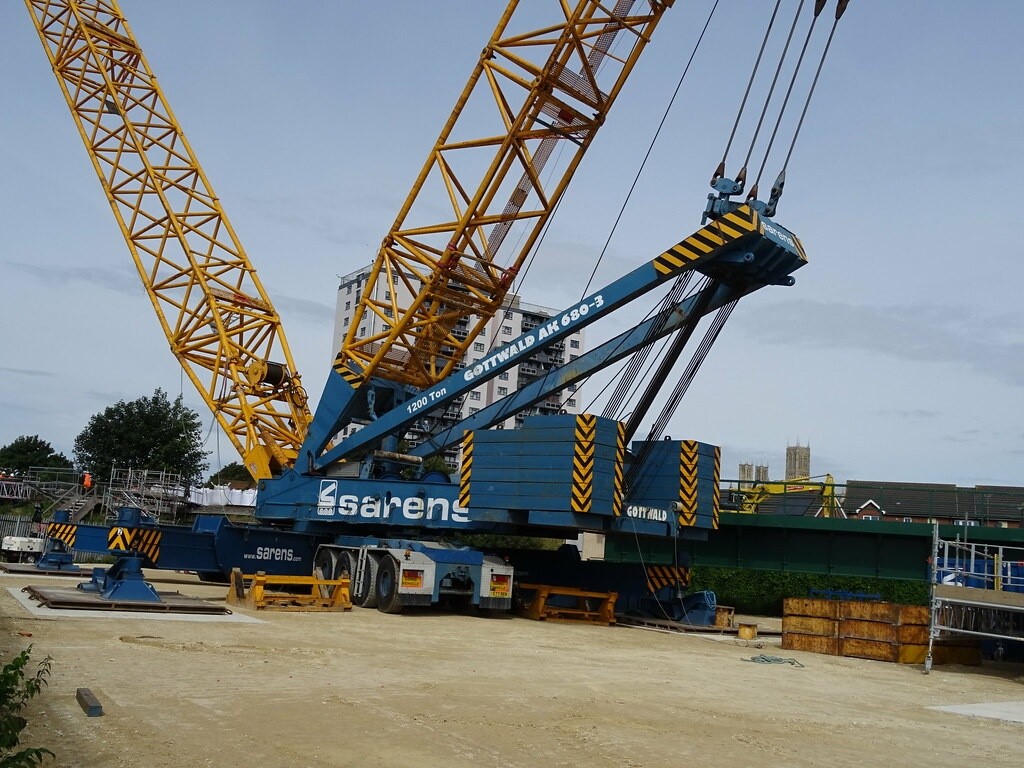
[719,471,837,519]
[27,0,856,615]
